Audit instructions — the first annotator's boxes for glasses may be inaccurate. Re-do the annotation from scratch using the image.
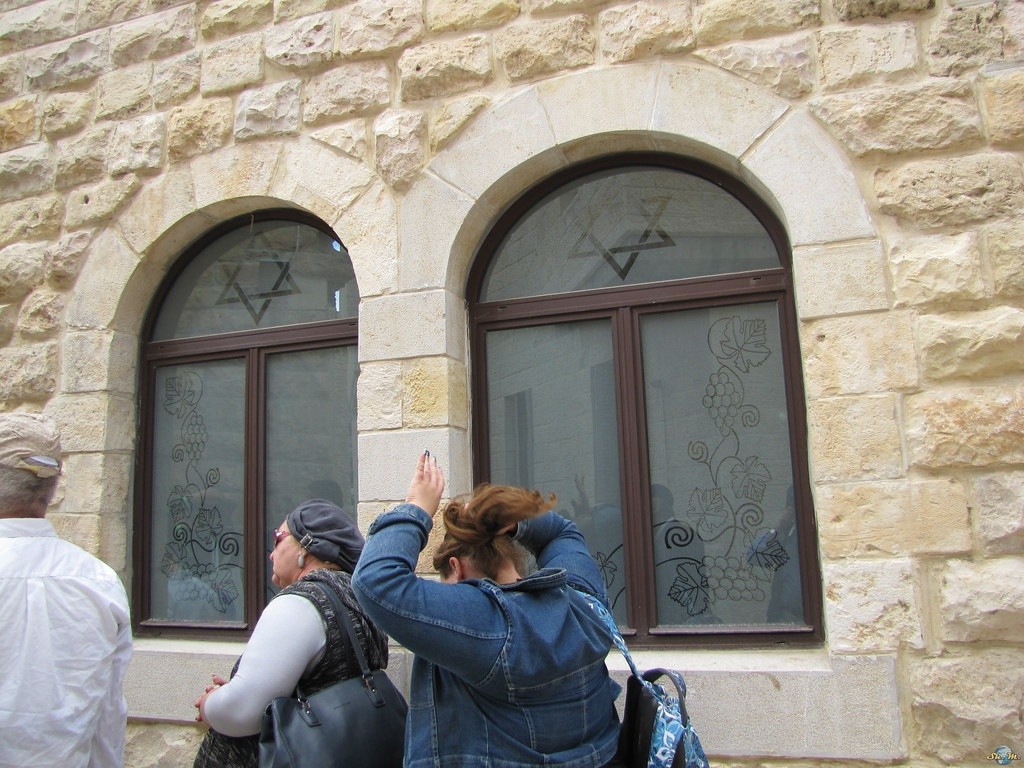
[274,528,291,547]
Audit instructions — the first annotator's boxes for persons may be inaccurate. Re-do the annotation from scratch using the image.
[2,409,134,768]
[151,478,343,622]
[189,495,390,768]
[351,449,622,768]
[556,473,806,624]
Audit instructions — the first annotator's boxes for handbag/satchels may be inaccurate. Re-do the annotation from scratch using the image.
[259,670,409,768]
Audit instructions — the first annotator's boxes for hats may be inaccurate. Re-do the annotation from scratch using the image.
[1,413,62,477]
[287,499,366,575]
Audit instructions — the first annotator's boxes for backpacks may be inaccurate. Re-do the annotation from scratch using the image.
[618,668,709,768]
